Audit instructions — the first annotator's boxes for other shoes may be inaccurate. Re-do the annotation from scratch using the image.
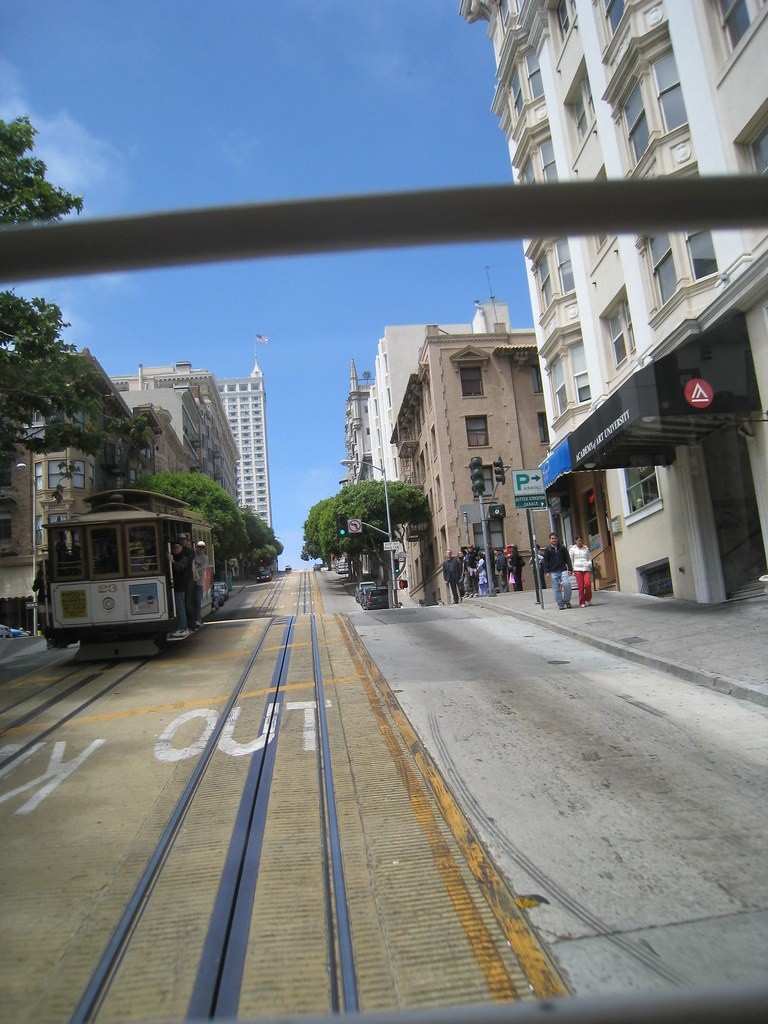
[587,600,592,605]
[566,603,571,608]
[171,630,189,637]
[473,594,479,599]
[461,592,469,598]
[467,594,473,599]
[580,604,586,608]
[559,604,564,610]
[196,621,203,628]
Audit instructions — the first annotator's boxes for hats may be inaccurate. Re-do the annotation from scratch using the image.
[510,544,517,549]
[179,534,187,539]
[197,541,206,546]
[495,548,503,551]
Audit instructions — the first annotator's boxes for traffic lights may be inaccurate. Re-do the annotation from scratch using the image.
[394,559,400,575]
[336,515,347,537]
[493,456,506,486]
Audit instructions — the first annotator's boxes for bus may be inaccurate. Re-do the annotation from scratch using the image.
[43,488,216,661]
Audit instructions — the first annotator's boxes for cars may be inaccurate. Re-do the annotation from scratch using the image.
[285,565,292,570]
[257,570,272,583]
[337,562,349,574]
[355,581,389,610]
[213,582,228,597]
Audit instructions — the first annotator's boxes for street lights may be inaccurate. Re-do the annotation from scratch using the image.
[339,460,398,608]
[16,462,39,636]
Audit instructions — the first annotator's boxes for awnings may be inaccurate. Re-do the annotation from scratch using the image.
[539,311,763,498]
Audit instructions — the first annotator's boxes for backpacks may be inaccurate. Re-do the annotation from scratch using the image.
[519,555,525,566]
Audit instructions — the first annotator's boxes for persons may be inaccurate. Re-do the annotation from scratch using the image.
[543,532,573,610]
[568,535,592,607]
[417,599,425,607]
[398,602,404,608]
[131,533,209,637]
[436,598,445,605]
[443,544,547,604]
[32,559,52,639]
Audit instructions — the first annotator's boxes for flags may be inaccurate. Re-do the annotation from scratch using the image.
[256,334,268,344]
